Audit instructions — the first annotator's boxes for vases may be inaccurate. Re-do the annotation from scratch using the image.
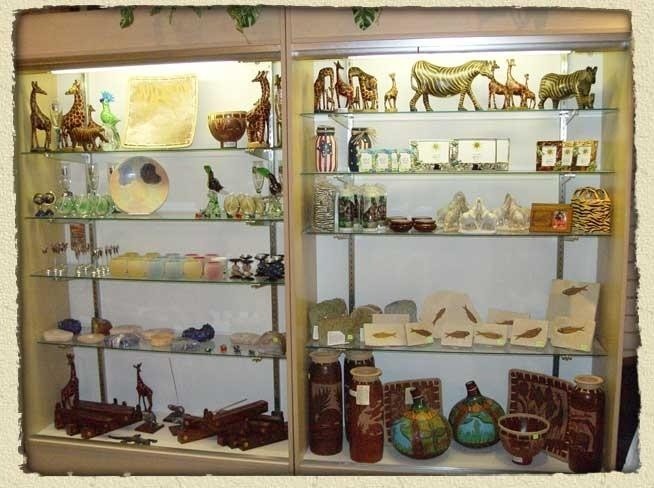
[308,348,506,464]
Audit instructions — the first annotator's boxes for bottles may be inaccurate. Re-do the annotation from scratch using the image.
[334,182,436,233]
[357,146,413,175]
[314,125,338,173]
[343,351,376,443]
[54,163,113,217]
[309,349,343,455]
[350,365,385,463]
[348,127,372,171]
[389,388,451,459]
[448,382,506,449]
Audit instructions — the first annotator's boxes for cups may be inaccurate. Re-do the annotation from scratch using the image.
[110,251,226,280]
[208,110,247,149]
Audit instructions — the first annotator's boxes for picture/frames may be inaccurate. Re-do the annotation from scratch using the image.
[536,141,598,171]
[529,204,572,233]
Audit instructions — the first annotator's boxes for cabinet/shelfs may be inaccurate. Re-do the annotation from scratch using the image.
[12,5,294,477]
[285,4,633,477]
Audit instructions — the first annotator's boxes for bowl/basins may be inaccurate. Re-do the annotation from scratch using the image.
[496,411,551,466]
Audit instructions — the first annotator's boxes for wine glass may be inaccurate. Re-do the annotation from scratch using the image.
[50,103,63,152]
[40,241,118,278]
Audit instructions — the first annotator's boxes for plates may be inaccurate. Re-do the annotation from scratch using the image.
[110,155,168,214]
